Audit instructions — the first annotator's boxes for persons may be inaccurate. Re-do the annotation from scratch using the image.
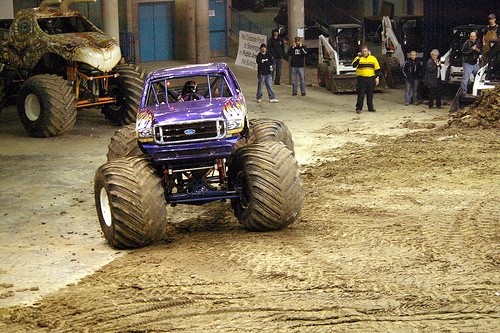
[489,25,500,80]
[287,36,308,96]
[403,51,424,105]
[462,32,480,93]
[479,14,499,47]
[424,49,444,109]
[177,80,205,102]
[352,46,381,114]
[268,29,284,85]
[256,44,279,103]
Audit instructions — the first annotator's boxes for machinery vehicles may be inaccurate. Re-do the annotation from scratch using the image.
[317,23,388,94]
[376,15,429,87]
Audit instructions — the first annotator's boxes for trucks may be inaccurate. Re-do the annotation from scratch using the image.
[447,24,489,88]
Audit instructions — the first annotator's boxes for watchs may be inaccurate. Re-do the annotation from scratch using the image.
[376,76,379,78]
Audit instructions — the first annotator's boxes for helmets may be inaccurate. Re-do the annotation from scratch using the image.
[184,81,198,95]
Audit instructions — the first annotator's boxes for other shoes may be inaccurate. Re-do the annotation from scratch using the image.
[368,108,376,112]
[406,102,409,106]
[292,93,297,96]
[414,102,419,105]
[302,93,305,96]
[270,99,279,103]
[257,99,261,102]
[357,110,361,113]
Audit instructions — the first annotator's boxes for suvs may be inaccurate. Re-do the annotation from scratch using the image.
[93,61,301,249]
[0,0,149,138]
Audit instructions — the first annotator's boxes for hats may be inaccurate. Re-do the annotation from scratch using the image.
[295,37,300,42]
[488,14,496,19]
[260,44,266,49]
